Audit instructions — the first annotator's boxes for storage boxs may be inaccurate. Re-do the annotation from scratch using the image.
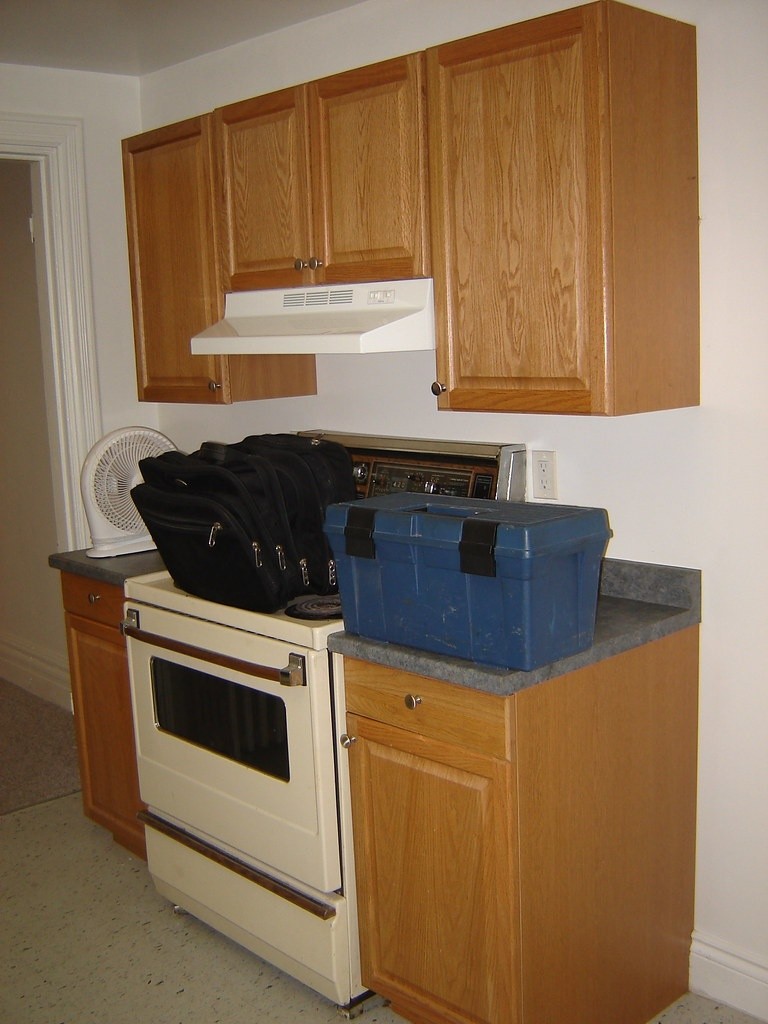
[323,492,615,672]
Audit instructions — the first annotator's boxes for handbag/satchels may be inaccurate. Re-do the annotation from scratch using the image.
[130,434,356,614]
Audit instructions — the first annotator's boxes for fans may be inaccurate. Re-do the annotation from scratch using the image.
[80,426,181,559]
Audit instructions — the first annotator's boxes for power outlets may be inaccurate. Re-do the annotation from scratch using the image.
[532,451,557,499]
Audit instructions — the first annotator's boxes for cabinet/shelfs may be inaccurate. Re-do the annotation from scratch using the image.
[340,626,699,1024]
[60,571,147,861]
[121,1,703,416]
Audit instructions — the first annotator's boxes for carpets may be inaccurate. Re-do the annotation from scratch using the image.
[0,677,80,816]
